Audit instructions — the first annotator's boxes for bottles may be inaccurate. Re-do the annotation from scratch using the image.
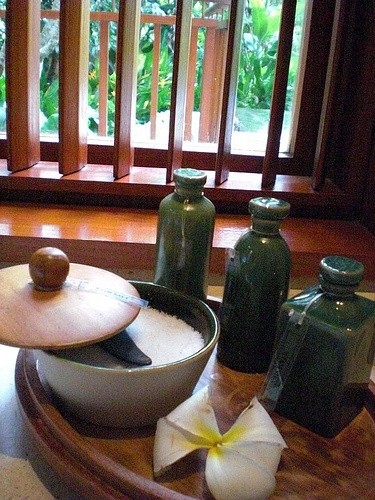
[260,255,375,437]
[217,197,291,375]
[154,167,216,302]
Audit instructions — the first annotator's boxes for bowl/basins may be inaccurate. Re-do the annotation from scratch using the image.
[33,281,221,429]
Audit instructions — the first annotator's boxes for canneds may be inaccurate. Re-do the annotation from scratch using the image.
[153,168,217,304]
[260,254,374,438]
[215,196,292,374]
